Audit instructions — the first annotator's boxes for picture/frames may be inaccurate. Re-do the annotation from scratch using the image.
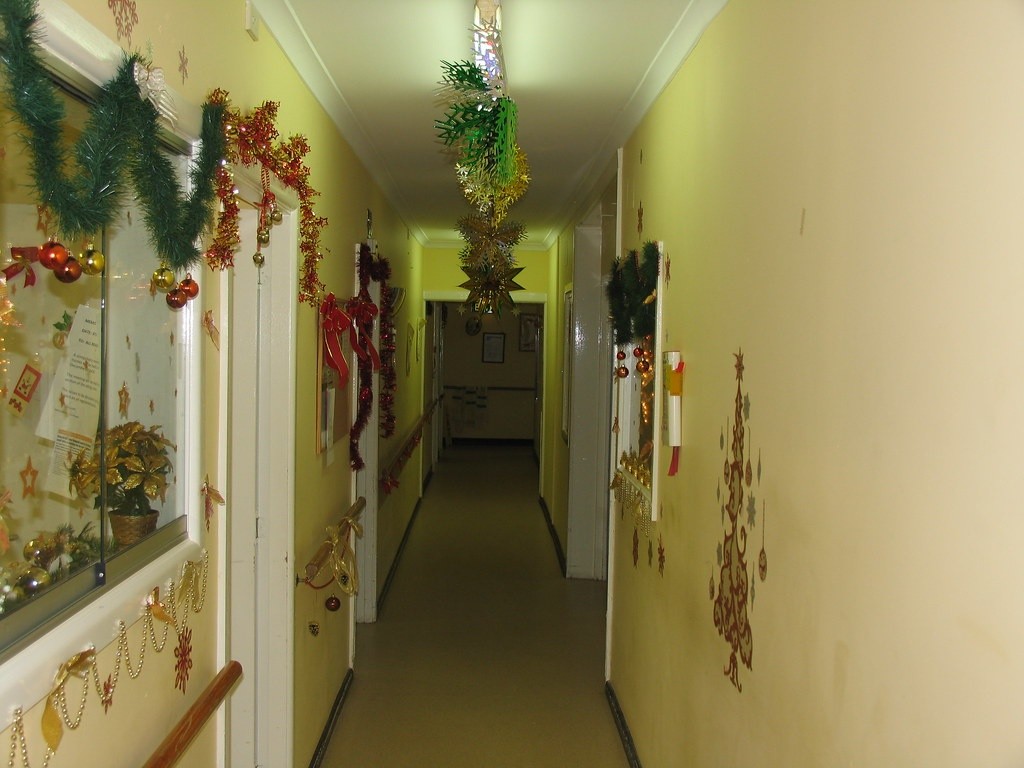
[482,332,505,364]
[518,312,539,352]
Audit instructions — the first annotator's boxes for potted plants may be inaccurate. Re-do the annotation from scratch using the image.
[68,422,174,545]
